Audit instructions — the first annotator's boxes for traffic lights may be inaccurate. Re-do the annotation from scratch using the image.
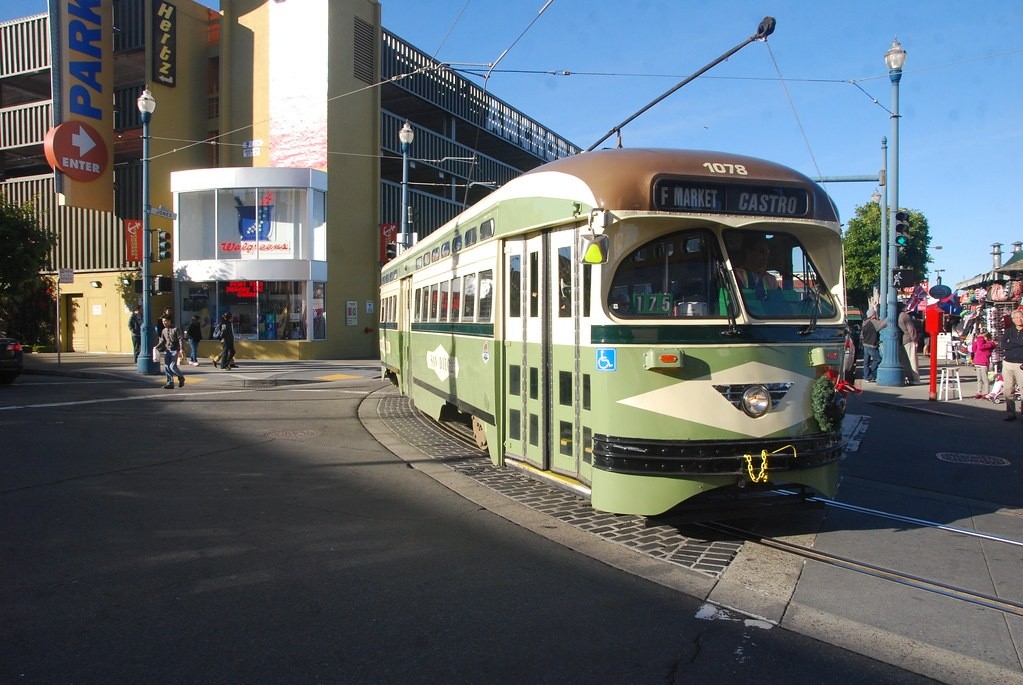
[892,269,915,288]
[157,230,170,260]
[155,274,172,294]
[386,243,396,260]
[895,211,910,247]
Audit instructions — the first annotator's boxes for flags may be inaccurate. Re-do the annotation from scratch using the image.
[904,283,928,313]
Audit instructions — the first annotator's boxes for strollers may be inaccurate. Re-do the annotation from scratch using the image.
[989,377,1021,403]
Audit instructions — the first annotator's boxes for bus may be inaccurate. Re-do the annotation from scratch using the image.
[378,147,848,525]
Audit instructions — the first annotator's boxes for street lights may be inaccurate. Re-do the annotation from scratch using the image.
[397,118,414,254]
[876,36,907,388]
[135,84,157,373]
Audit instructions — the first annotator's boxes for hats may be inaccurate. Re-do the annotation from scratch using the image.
[898,302,906,309]
[975,328,989,336]
[866,309,876,318]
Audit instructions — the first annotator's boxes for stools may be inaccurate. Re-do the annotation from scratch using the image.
[939,367,963,401]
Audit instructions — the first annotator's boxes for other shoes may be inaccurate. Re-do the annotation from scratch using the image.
[866,379,876,382]
[212,359,217,367]
[163,384,174,388]
[221,366,231,369]
[976,394,987,399]
[179,378,185,387]
[909,381,923,384]
[189,361,199,366]
[1004,415,1017,421]
[231,364,239,368]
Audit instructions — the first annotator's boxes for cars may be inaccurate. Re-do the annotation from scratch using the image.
[845,327,857,385]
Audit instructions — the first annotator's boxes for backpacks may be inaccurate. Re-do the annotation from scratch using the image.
[213,324,222,339]
[183,324,189,340]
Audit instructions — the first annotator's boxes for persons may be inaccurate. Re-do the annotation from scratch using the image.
[312,307,326,338]
[860,302,1023,421]
[721,234,781,297]
[128,305,239,389]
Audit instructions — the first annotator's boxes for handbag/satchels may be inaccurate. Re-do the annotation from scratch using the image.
[178,352,187,365]
[153,348,160,362]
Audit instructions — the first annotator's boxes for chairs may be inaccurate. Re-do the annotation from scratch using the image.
[613,287,630,306]
[665,280,690,303]
[633,284,652,295]
